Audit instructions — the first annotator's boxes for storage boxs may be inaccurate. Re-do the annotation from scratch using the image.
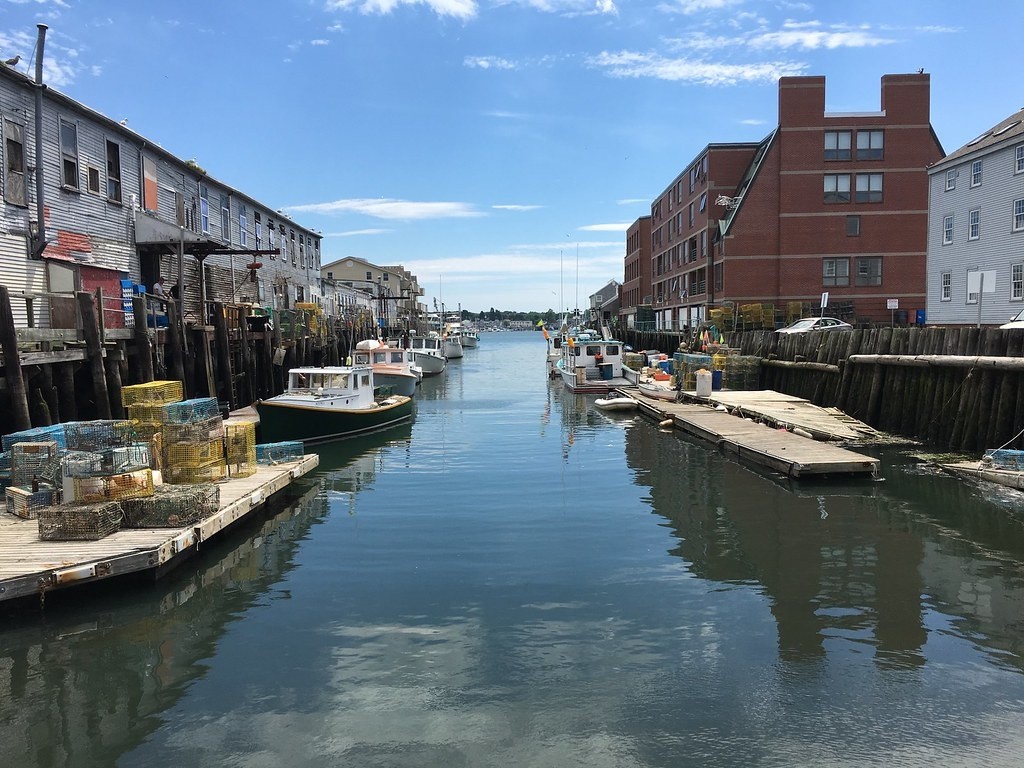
[0,379,304,548]
[623,351,726,391]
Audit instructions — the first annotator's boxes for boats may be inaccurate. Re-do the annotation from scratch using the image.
[441,335,464,358]
[259,363,412,416]
[397,329,449,375]
[351,337,415,401]
[460,329,481,348]
[544,324,640,396]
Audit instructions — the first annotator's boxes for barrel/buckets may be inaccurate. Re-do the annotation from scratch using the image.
[916,309,926,326]
[659,362,670,373]
[695,372,712,397]
[712,370,722,390]
[602,364,613,380]
[575,366,586,385]
[896,310,908,326]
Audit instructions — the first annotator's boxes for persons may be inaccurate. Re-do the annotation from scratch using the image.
[168,278,195,326]
[153,277,169,311]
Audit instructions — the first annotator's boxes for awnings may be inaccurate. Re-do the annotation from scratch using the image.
[135,210,229,252]
